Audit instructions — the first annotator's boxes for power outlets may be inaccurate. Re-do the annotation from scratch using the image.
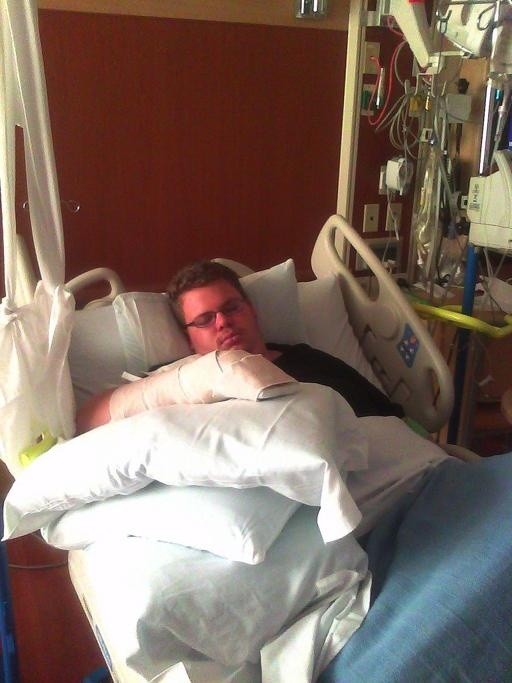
[363,203,402,233]
[364,83,377,118]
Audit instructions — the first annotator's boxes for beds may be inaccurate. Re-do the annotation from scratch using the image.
[26,248,512,682]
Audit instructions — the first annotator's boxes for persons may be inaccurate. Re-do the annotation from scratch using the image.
[66,252,409,437]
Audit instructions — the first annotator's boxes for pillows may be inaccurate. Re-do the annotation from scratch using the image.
[38,487,295,566]
[73,508,374,681]
[111,259,305,381]
[1,385,366,549]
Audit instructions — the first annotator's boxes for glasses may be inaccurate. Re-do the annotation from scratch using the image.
[183,296,247,329]
